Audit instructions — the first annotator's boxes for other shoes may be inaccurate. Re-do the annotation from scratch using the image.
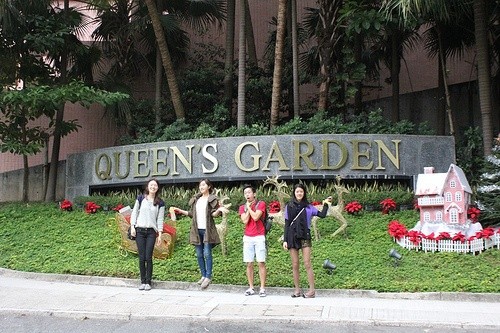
[139,283,146,290]
[303,292,315,298]
[145,284,151,291]
[200,279,210,288]
[292,293,300,297]
[258,288,266,297]
[197,277,204,285]
[244,288,256,296]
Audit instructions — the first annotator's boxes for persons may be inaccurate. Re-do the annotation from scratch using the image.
[239,186,267,297]
[129,179,166,291]
[173,178,229,288]
[282,184,332,299]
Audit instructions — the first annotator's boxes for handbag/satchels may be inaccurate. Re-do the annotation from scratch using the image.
[127,225,137,240]
